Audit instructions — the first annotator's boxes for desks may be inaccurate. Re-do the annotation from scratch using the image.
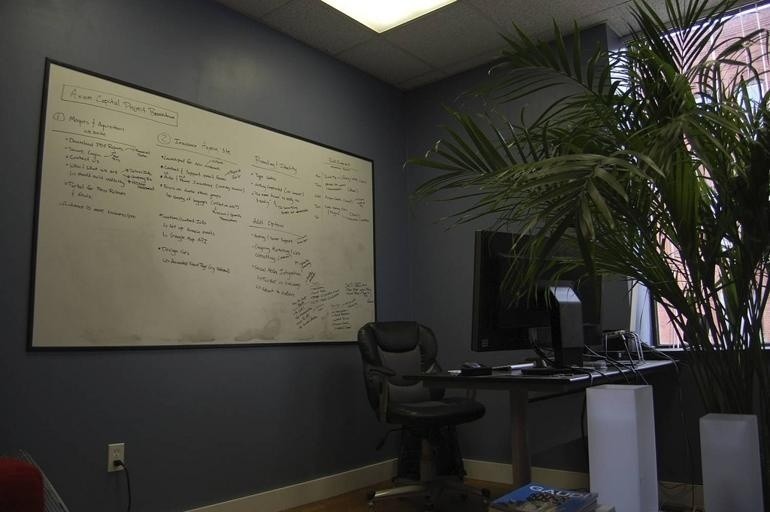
[400,359,679,490]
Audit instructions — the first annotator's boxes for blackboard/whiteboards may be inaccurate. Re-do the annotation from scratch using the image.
[26,55,377,353]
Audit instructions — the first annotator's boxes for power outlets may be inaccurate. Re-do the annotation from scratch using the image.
[106,443,125,473]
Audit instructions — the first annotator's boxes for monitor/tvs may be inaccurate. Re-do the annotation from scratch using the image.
[472,232,603,371]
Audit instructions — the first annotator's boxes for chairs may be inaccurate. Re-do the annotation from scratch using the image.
[357,321,489,512]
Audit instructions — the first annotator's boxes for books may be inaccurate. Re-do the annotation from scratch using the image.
[487,480,617,512]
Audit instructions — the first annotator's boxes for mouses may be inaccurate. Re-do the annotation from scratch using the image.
[461,360,482,372]
